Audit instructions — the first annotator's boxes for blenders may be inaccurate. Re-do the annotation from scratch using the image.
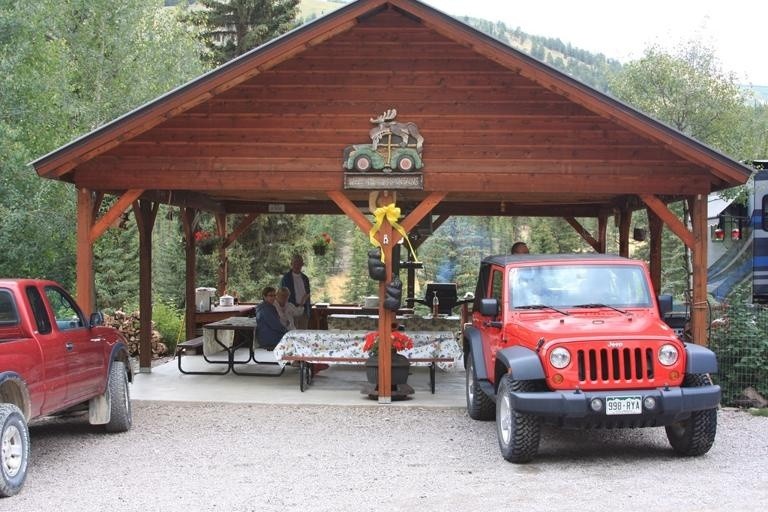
[196,287,216,313]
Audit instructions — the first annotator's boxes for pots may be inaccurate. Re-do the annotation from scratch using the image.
[219,294,234,306]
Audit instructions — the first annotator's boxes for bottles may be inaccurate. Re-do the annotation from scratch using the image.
[200,300,205,312]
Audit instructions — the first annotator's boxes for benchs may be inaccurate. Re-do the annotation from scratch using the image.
[178,335,204,375]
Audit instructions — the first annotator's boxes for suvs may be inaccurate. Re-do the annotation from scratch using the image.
[463,253,721,463]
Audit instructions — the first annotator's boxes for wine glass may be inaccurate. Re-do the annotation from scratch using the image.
[432,291,439,305]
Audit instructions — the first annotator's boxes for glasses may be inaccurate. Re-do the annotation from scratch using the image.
[264,294,277,297]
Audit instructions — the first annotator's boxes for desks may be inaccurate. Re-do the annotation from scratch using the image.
[284,313,461,393]
[204,316,257,374]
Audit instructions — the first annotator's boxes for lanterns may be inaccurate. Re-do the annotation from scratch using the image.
[714,228,724,241]
[732,227,742,240]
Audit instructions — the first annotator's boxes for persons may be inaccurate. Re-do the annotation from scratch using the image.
[254,254,312,350]
[511,242,530,255]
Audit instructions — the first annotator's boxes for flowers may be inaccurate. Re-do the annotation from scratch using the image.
[312,232,335,256]
[195,230,224,255]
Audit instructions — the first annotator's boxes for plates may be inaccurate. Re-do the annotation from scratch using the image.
[315,302,330,308]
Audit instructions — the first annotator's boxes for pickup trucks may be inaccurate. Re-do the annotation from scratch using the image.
[0,278,133,497]
